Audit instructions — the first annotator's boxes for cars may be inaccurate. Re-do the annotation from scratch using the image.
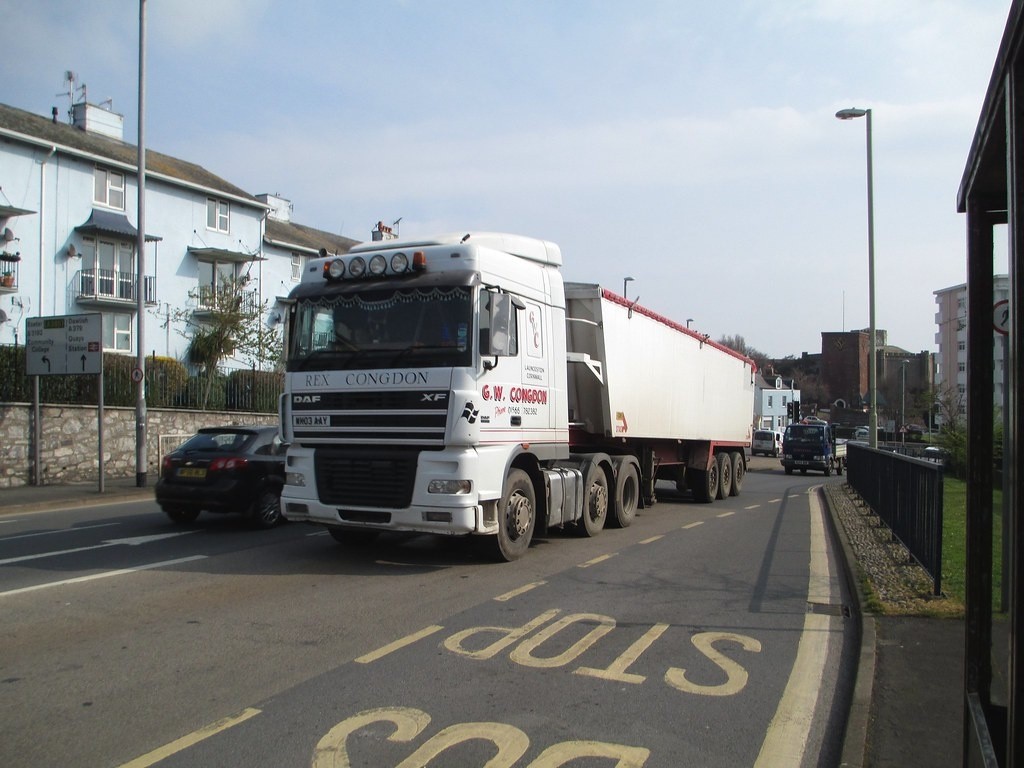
[803,416,827,424]
[854,425,869,440]
[154,424,290,529]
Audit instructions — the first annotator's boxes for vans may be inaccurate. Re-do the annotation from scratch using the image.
[751,429,783,456]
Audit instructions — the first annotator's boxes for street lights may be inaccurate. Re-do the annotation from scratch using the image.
[901,357,911,447]
[623,277,636,298]
[686,319,693,328]
[790,379,796,423]
[835,103,881,449]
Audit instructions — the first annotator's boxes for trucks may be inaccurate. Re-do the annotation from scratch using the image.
[780,421,847,476]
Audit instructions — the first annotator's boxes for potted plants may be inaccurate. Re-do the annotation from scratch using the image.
[0,270,16,287]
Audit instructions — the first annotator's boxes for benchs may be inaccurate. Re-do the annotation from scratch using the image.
[206,440,243,450]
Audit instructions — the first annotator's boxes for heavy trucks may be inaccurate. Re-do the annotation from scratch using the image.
[276,220,758,563]
